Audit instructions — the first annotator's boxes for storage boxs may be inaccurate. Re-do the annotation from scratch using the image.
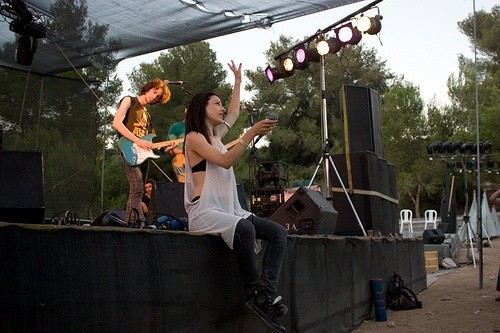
[424,250,439,273]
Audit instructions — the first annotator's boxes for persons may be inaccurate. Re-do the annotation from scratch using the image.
[182,59,289,333]
[166,104,187,184]
[111,76,180,228]
[140,178,188,231]
[488,188,500,302]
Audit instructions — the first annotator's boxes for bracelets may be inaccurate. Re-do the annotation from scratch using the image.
[239,138,249,148]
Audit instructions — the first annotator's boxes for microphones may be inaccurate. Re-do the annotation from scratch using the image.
[164,79,187,85]
[253,114,277,142]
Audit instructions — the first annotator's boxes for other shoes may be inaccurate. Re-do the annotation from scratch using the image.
[243,287,289,333]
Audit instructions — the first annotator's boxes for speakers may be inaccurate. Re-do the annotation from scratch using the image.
[267,188,338,236]
[328,84,400,237]
[422,229,445,243]
[0,150,45,224]
[150,179,186,224]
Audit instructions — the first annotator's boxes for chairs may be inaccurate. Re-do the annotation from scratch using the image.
[400,209,414,233]
[424,209,437,231]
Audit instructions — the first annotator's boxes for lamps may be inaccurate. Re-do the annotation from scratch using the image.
[265,5,383,84]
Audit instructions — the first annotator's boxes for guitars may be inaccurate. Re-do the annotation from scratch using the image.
[172,137,241,184]
[117,133,186,166]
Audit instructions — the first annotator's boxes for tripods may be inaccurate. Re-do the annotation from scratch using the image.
[451,161,480,269]
[307,37,368,236]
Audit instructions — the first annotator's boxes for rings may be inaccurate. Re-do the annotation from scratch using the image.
[265,119,269,124]
